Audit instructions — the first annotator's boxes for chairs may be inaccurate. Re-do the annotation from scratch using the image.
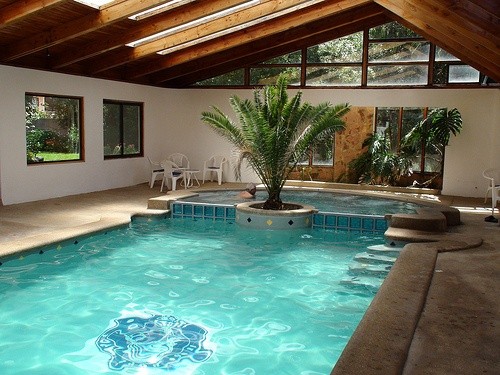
[202,152,228,185]
[482,168,500,216]
[147,154,201,193]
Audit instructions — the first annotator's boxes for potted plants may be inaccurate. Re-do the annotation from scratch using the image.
[201,75,352,232]
